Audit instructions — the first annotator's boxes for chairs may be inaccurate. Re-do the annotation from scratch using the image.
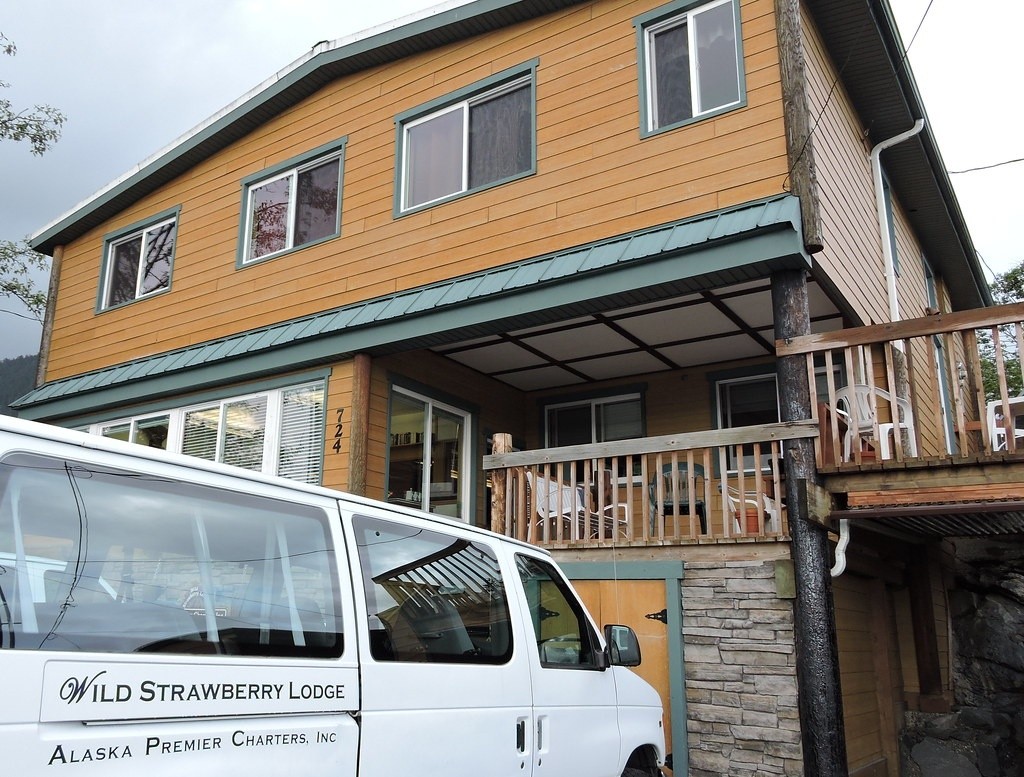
[391,592,477,654]
[987,391,1024,451]
[835,384,918,463]
[718,484,785,535]
[648,462,707,537]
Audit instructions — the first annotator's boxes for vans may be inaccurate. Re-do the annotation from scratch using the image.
[0,414,666,777]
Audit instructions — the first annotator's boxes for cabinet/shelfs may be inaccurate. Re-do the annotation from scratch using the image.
[388,437,458,517]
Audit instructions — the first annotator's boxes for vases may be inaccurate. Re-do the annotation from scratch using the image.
[850,451,876,462]
[735,508,766,533]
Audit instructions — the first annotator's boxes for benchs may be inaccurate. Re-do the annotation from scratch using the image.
[526,471,628,539]
